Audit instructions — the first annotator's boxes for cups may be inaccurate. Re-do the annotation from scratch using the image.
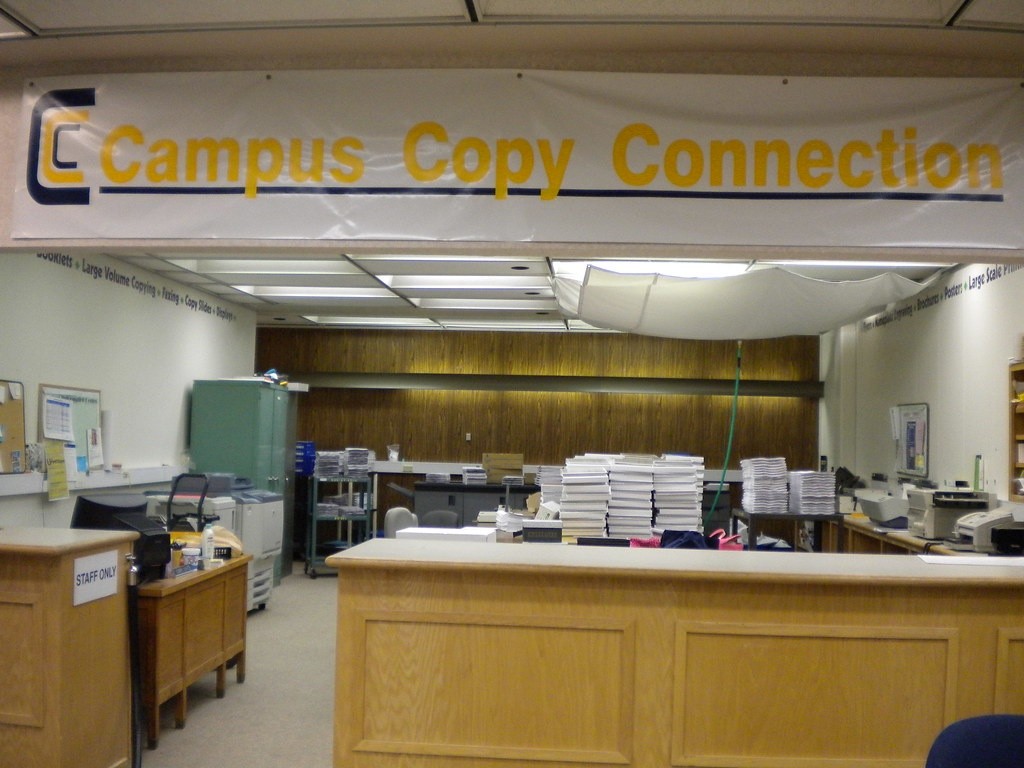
[182,548,200,572]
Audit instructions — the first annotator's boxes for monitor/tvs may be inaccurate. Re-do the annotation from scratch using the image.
[72,493,172,582]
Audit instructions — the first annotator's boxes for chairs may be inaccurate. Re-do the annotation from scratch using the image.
[925,714,1022,767]
[385,508,415,540]
[421,511,460,531]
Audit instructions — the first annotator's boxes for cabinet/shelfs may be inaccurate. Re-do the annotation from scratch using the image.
[192,379,289,589]
[1006,363,1024,502]
[307,478,373,577]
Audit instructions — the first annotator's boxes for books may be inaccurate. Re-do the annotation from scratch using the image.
[315,448,369,517]
[740,457,836,516]
[427,452,706,543]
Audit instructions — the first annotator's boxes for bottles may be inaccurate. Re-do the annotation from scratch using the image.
[202,522,216,561]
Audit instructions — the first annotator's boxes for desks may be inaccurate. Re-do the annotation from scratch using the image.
[732,508,845,551]
[131,554,255,748]
[0,527,135,768]
[322,515,1024,768]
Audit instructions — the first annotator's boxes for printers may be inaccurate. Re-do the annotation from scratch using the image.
[143,471,283,614]
[858,481,1024,556]
